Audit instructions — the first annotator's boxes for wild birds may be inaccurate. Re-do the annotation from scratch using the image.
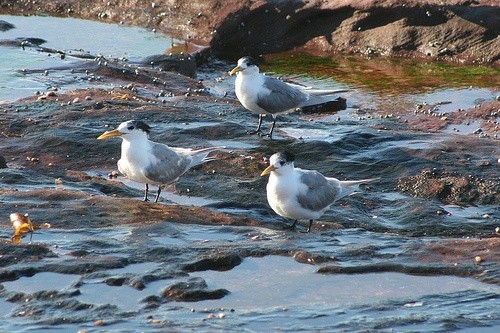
[260,148,382,235]
[8,212,52,247]
[94,118,227,206]
[227,56,348,138]
